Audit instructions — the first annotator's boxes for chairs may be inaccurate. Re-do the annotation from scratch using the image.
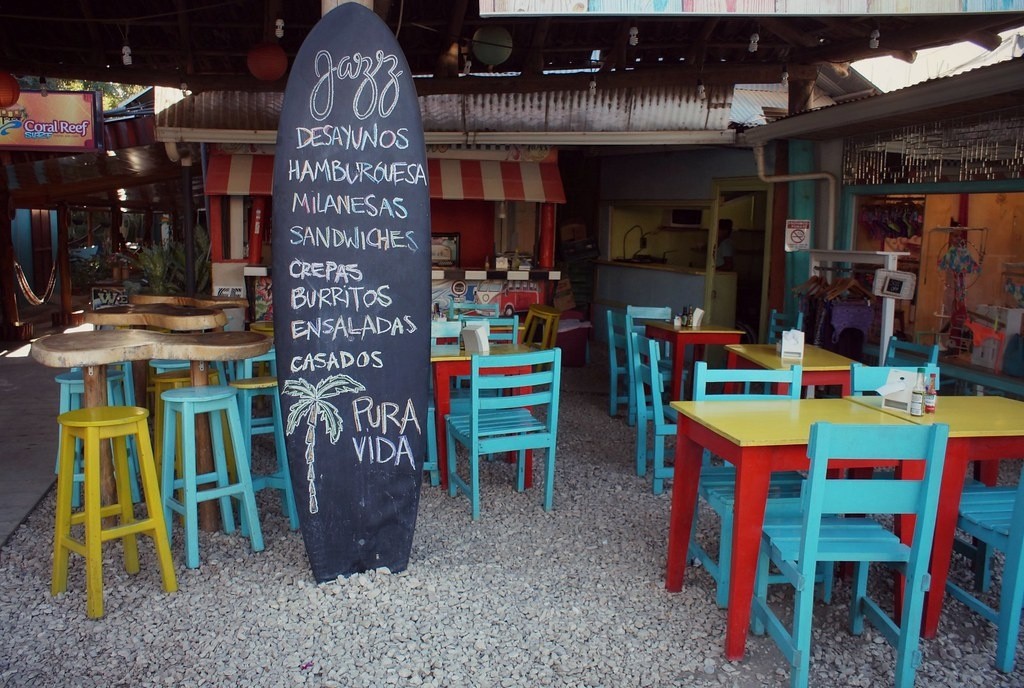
[850,362,994,592]
[748,422,950,688]
[422,299,561,523]
[627,305,673,367]
[606,310,688,424]
[686,361,839,609]
[884,335,939,367]
[945,467,1024,673]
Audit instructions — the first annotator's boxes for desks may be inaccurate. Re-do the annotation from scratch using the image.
[844,395,1024,639]
[665,399,926,662]
[31,327,274,533]
[723,341,866,399]
[435,322,525,344]
[128,292,250,333]
[645,321,747,402]
[430,343,533,489]
[85,304,228,401]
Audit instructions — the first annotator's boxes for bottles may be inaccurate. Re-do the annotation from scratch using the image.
[511,247,521,269]
[484,255,490,267]
[925,373,937,414]
[686,304,693,328]
[681,306,688,327]
[910,368,927,416]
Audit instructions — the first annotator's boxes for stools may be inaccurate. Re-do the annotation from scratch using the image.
[52,321,302,619]
[520,304,562,372]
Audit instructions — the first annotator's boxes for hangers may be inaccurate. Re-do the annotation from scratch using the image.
[791,265,877,308]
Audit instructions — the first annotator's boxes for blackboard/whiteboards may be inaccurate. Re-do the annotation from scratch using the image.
[273,0,435,583]
